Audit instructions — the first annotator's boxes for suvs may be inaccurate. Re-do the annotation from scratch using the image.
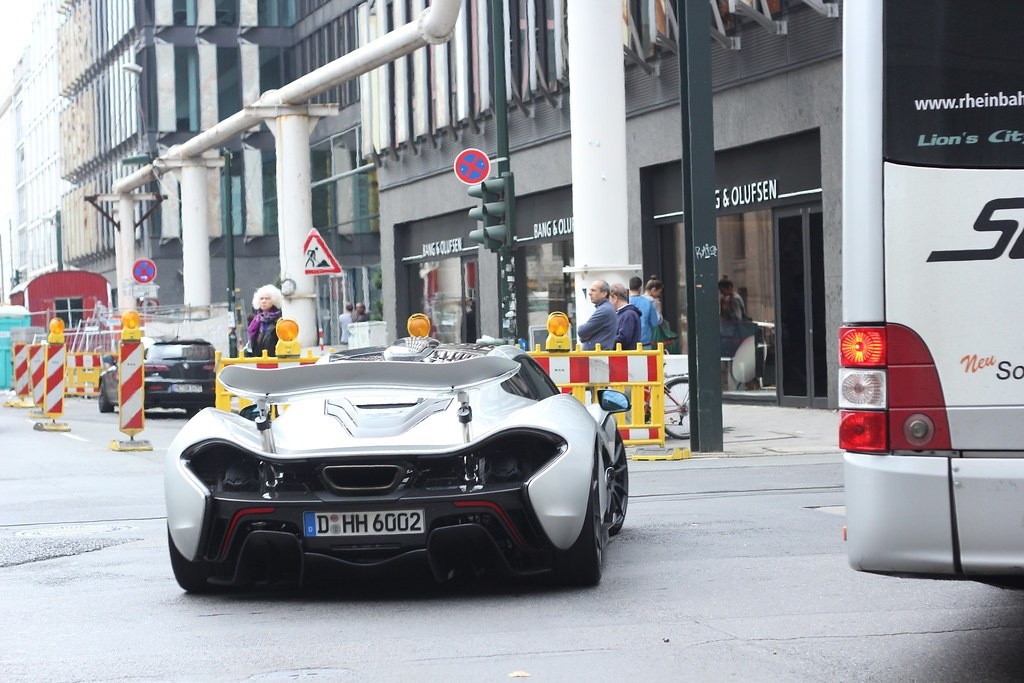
[97,336,224,417]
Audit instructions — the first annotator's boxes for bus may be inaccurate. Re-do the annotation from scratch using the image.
[838,1,1024,597]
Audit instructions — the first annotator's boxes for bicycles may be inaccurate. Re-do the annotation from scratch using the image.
[663,360,695,442]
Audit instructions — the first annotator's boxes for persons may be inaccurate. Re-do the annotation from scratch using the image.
[577,279,617,350]
[608,282,642,350]
[247,285,284,357]
[338,303,353,344]
[460,300,480,343]
[644,275,664,324]
[718,274,747,321]
[628,277,658,350]
[350,303,369,322]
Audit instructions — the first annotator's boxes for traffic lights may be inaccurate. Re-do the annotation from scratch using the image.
[469,177,498,252]
[481,172,518,249]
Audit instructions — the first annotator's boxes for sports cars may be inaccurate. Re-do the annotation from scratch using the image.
[162,337,633,597]
